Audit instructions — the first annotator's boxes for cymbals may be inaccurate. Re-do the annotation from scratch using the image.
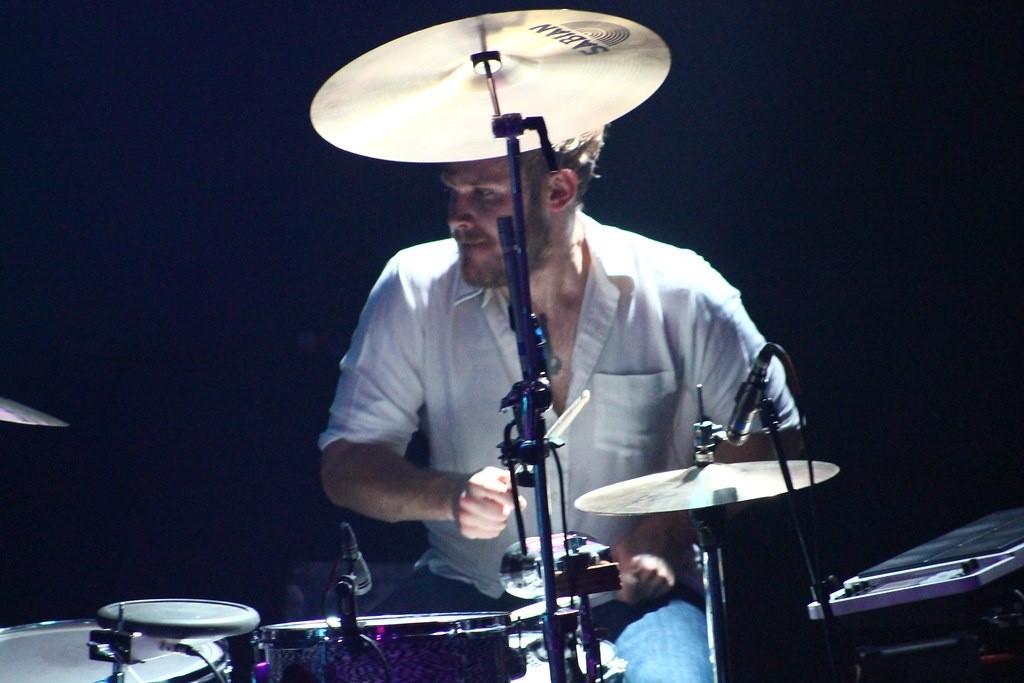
[573,458,841,516]
[1,395,71,432]
[309,6,673,165]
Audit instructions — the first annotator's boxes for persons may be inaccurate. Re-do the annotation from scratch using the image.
[319,126,801,683]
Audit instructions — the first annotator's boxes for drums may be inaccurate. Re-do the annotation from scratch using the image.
[259,610,515,683]
[0,617,235,683]
[97,596,263,638]
